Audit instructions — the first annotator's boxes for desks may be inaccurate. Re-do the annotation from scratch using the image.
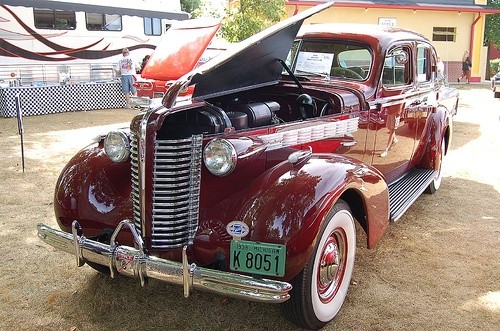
[0,81,137,118]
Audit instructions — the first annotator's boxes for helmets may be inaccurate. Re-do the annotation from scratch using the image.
[122,48,129,53]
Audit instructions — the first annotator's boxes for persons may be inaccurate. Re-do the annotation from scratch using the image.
[379,96,404,157]
[112,48,141,109]
[458,50,472,85]
[140,55,152,74]
[437,56,444,83]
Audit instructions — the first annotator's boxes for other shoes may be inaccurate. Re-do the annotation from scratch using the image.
[380,149,387,157]
[389,138,398,150]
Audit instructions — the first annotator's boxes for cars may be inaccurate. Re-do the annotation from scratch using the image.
[36,2,454,331]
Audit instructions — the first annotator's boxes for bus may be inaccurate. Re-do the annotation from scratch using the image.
[0,0,191,88]
